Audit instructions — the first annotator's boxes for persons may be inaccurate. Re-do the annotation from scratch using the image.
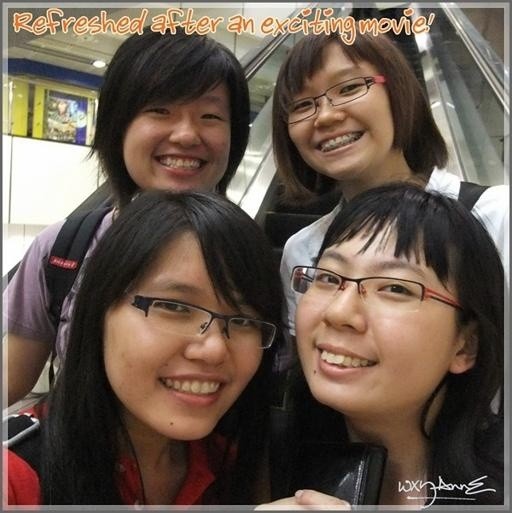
[268,20,506,445]
[2,188,289,513]
[252,180,504,513]
[2,24,250,427]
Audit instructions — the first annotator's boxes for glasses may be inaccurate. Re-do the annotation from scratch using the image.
[123,289,278,348]
[283,76,387,125]
[290,266,462,312]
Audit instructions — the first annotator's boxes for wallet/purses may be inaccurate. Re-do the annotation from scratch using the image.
[292,441,386,505]
